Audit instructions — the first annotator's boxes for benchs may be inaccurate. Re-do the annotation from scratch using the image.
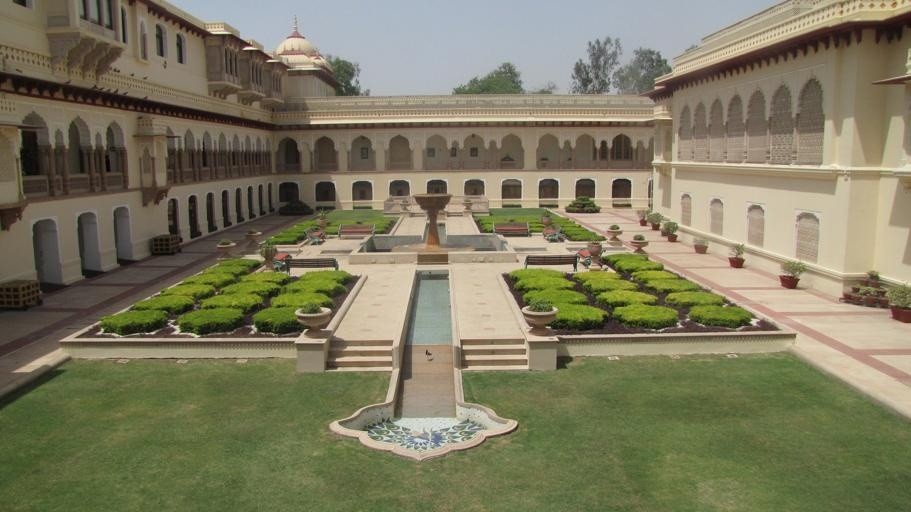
[493,221,531,237]
[304,225,328,246]
[524,254,578,272]
[284,257,340,276]
[338,223,377,240]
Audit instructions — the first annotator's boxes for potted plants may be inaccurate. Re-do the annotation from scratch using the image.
[541,210,553,226]
[314,209,330,227]
[778,259,806,289]
[217,228,277,270]
[840,271,911,323]
[521,299,559,336]
[586,209,680,256]
[694,236,710,254]
[728,243,746,269]
[295,300,333,339]
